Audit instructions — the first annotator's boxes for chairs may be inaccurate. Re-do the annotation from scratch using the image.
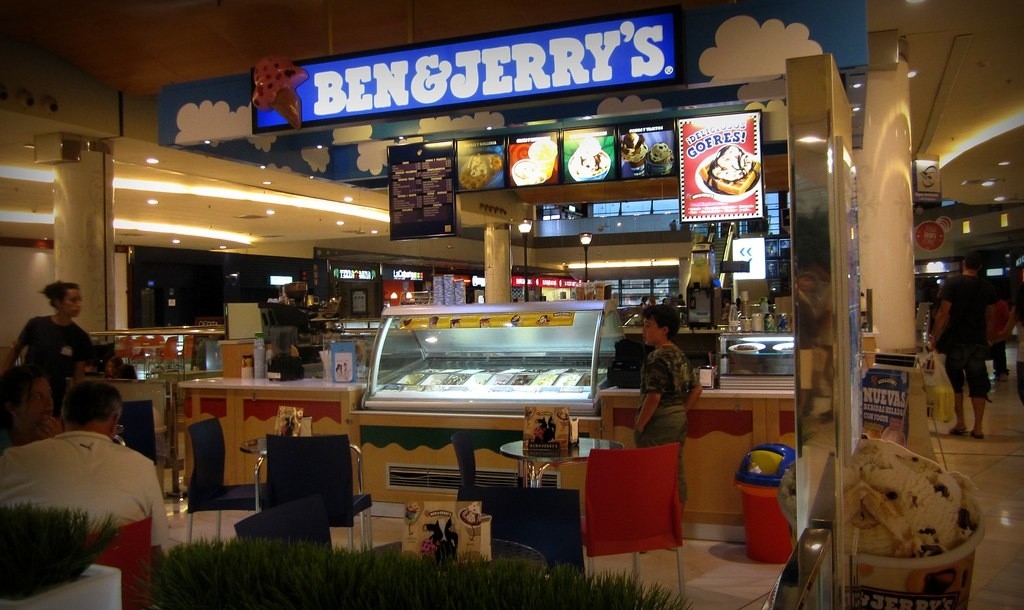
[107,335,194,359]
[579,441,687,605]
[450,431,492,514]
[185,416,265,545]
[114,398,158,469]
[456,484,585,575]
[234,494,333,554]
[264,433,374,551]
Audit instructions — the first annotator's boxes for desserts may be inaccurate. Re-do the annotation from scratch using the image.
[698,144,760,196]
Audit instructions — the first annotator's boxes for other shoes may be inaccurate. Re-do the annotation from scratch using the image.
[995,372,1008,381]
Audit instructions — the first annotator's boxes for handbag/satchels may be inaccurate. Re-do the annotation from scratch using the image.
[917,350,956,423]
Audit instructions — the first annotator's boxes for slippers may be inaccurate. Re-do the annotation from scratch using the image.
[949,428,984,439]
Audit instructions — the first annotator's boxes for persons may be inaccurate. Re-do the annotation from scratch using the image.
[1,280,172,569]
[632,304,704,515]
[926,249,1024,438]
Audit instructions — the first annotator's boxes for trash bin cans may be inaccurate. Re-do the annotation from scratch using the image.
[734,443,796,565]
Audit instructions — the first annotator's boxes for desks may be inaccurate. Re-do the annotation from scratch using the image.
[239,432,366,551]
[360,539,547,572]
[500,438,625,487]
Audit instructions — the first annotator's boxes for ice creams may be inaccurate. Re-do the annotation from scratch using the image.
[460,153,502,190]
[568,137,610,180]
[620,132,674,178]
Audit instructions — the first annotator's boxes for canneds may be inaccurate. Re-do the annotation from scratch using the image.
[739,315,752,331]
[776,313,788,331]
[751,312,763,331]
[764,313,776,331]
